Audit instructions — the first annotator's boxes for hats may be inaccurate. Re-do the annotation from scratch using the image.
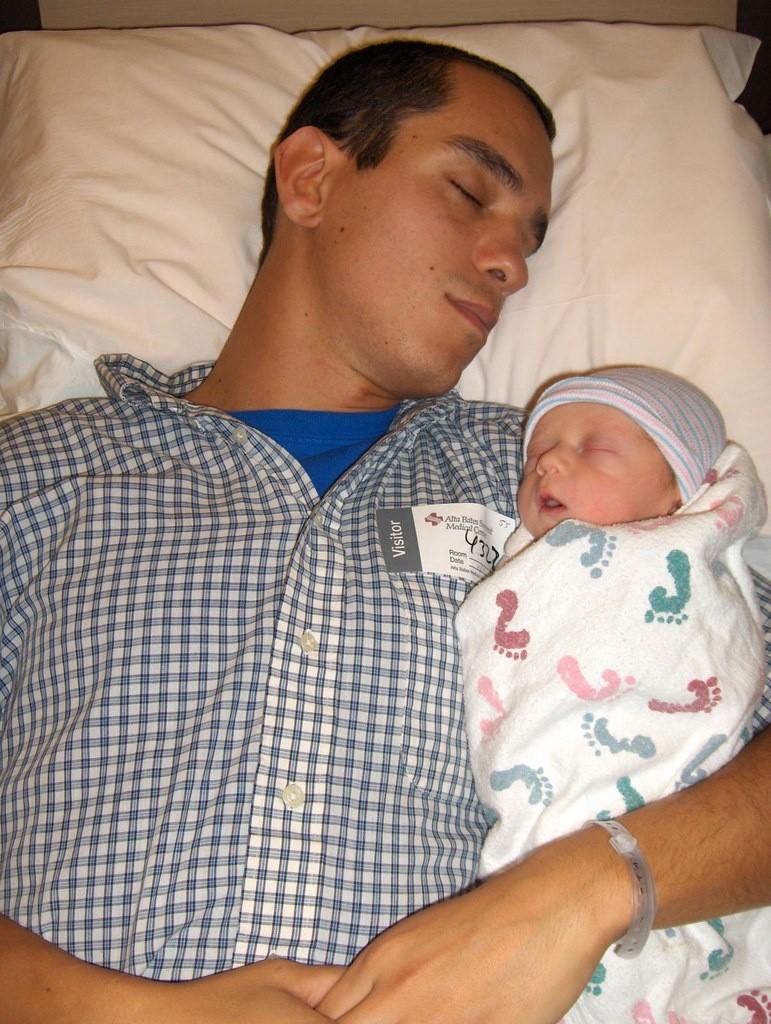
[522,366,727,506]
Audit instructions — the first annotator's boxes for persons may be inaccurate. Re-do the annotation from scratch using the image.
[0,38,771,1024]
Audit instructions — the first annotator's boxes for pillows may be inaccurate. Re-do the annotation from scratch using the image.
[0,24,771,535]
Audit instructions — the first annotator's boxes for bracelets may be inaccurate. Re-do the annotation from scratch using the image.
[581,820,658,960]
